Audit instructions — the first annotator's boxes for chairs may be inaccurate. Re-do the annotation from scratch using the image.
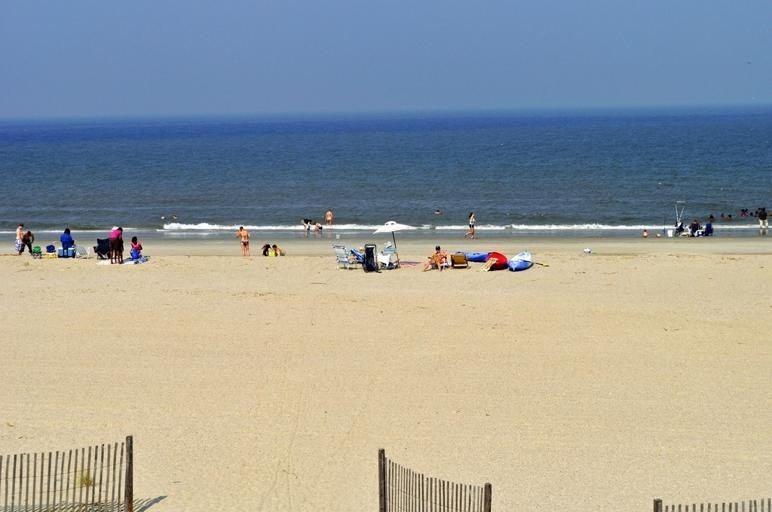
[452,254,468,268]
[332,244,398,273]
[93,239,109,259]
[432,251,447,268]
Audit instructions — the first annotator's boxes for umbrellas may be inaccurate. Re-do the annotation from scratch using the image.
[374,219,417,265]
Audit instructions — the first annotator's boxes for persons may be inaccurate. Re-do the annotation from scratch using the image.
[423,245,448,272]
[60,228,75,250]
[15,223,34,256]
[464,212,476,240]
[236,226,250,256]
[263,245,285,256]
[301,207,333,231]
[691,208,772,237]
[109,227,124,265]
[384,241,399,257]
[130,236,143,260]
[643,229,648,238]
